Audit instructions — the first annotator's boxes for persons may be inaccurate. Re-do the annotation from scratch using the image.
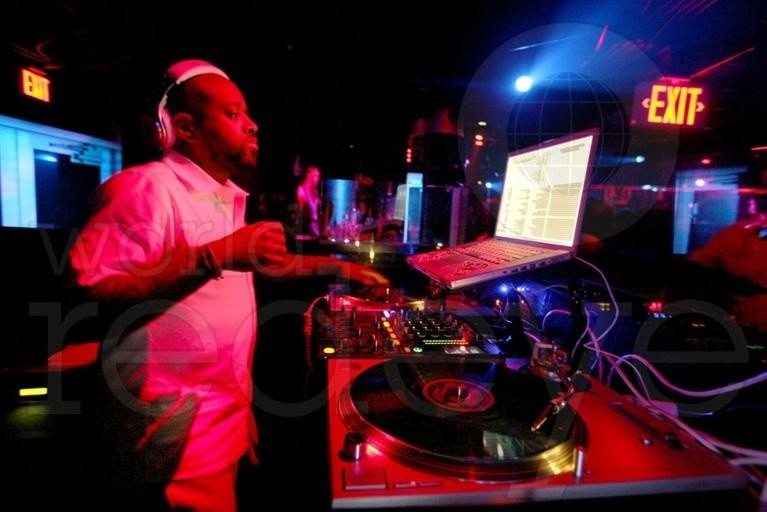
[61,60,391,512]
[740,195,758,219]
[354,166,383,242]
[297,167,322,237]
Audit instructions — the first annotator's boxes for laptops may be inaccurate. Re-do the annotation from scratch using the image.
[405,127,600,290]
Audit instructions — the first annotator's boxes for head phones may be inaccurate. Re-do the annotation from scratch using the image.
[155,68,232,152]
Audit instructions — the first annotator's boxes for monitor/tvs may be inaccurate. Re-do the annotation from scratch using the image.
[667,163,751,260]
[1,110,124,232]
